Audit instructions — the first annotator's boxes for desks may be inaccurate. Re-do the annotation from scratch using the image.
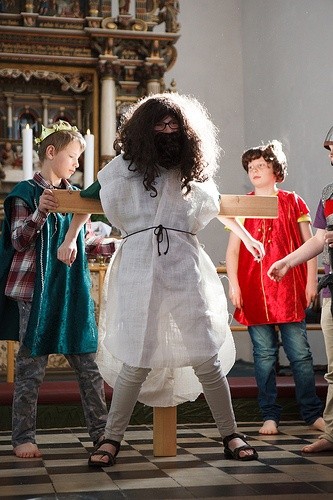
[0,263,108,380]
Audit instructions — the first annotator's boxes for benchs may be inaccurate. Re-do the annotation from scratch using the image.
[215,266,325,331]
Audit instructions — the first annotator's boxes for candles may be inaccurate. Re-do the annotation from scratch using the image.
[84,129,94,189]
[21,124,33,179]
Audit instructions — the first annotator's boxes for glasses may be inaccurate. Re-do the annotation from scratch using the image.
[153,119,180,131]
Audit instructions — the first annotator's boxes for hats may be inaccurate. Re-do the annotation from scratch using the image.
[323,125,333,151]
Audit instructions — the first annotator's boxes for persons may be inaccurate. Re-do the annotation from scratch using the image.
[267,127,333,454]
[226,141,325,435]
[56,94,265,467]
[3,121,120,459]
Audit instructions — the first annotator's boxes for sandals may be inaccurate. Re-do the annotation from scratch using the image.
[88,438,121,467]
[222,432,259,461]
